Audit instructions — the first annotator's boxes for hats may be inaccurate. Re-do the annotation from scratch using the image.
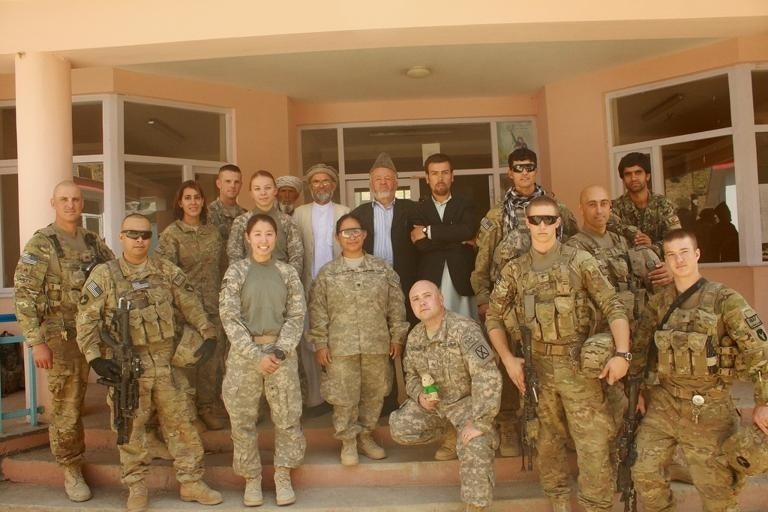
[369,151,398,174]
[305,163,341,187]
[275,175,303,195]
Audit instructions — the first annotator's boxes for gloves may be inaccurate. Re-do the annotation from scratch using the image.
[191,338,217,370]
[90,357,122,383]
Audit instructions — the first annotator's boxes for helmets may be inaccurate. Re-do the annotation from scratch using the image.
[170,322,205,370]
[627,244,663,279]
[720,424,768,476]
[579,334,617,380]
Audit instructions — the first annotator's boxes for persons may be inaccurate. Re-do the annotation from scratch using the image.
[157,180,226,433]
[389,278,502,511]
[75,211,223,511]
[472,150,768,512]
[218,215,306,506]
[306,214,411,467]
[218,153,502,415]
[16,181,174,503]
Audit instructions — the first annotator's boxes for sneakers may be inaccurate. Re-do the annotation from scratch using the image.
[190,419,207,433]
[499,425,521,457]
[243,474,264,507]
[434,436,458,461]
[127,479,149,512]
[63,467,92,502]
[201,411,226,430]
[273,473,296,506]
[341,442,359,465]
[145,432,176,461]
[358,438,388,461]
[180,479,223,506]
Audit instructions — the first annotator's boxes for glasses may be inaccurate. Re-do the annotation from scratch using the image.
[120,230,154,240]
[511,163,537,173]
[338,227,363,239]
[310,179,334,188]
[526,214,560,226]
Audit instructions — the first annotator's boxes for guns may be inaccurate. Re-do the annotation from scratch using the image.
[96,297,142,446]
[519,327,537,469]
[616,373,642,512]
